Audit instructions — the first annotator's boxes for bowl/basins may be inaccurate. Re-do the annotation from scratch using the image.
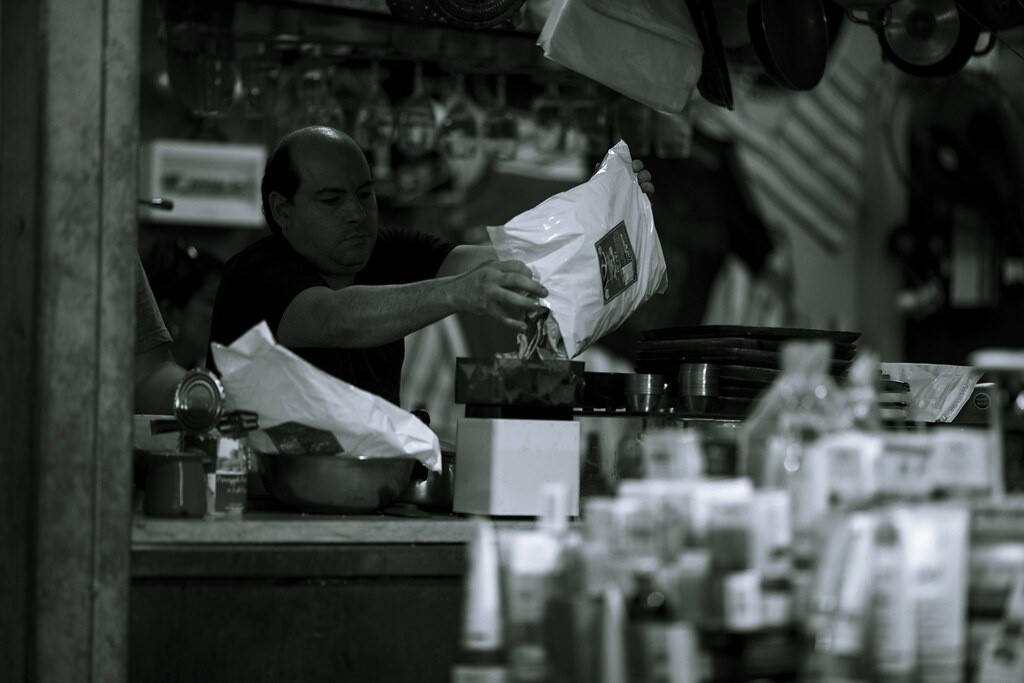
[253,451,416,516]
[381,452,459,517]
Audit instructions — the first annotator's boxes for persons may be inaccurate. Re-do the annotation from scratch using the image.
[195,125,656,407]
[135,253,190,410]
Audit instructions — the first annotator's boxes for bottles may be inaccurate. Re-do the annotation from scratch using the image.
[213,431,247,516]
[160,39,693,184]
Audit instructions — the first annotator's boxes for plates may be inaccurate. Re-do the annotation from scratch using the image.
[617,323,864,415]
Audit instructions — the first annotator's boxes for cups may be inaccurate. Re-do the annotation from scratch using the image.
[141,452,207,519]
